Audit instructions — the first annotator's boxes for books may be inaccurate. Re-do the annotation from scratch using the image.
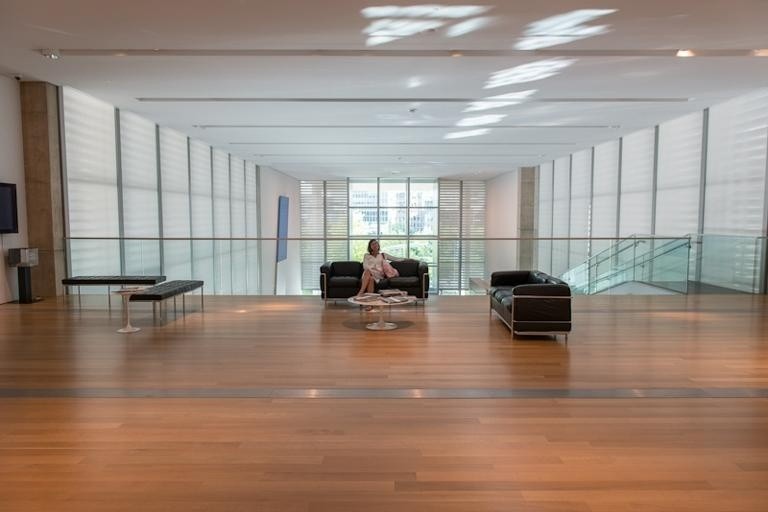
[379,289,408,303]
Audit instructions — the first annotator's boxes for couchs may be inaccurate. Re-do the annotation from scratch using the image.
[488,270,573,345]
[319,258,430,310]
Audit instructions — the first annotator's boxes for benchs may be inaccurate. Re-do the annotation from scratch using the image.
[60,276,165,317]
[130,279,206,329]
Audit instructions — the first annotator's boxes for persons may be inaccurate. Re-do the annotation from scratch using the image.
[357,239,415,311]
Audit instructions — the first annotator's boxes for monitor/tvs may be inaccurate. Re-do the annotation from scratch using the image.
[0,183,19,234]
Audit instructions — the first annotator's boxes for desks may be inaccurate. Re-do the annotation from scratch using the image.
[110,287,145,333]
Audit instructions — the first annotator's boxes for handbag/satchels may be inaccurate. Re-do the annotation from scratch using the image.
[382,262,399,278]
